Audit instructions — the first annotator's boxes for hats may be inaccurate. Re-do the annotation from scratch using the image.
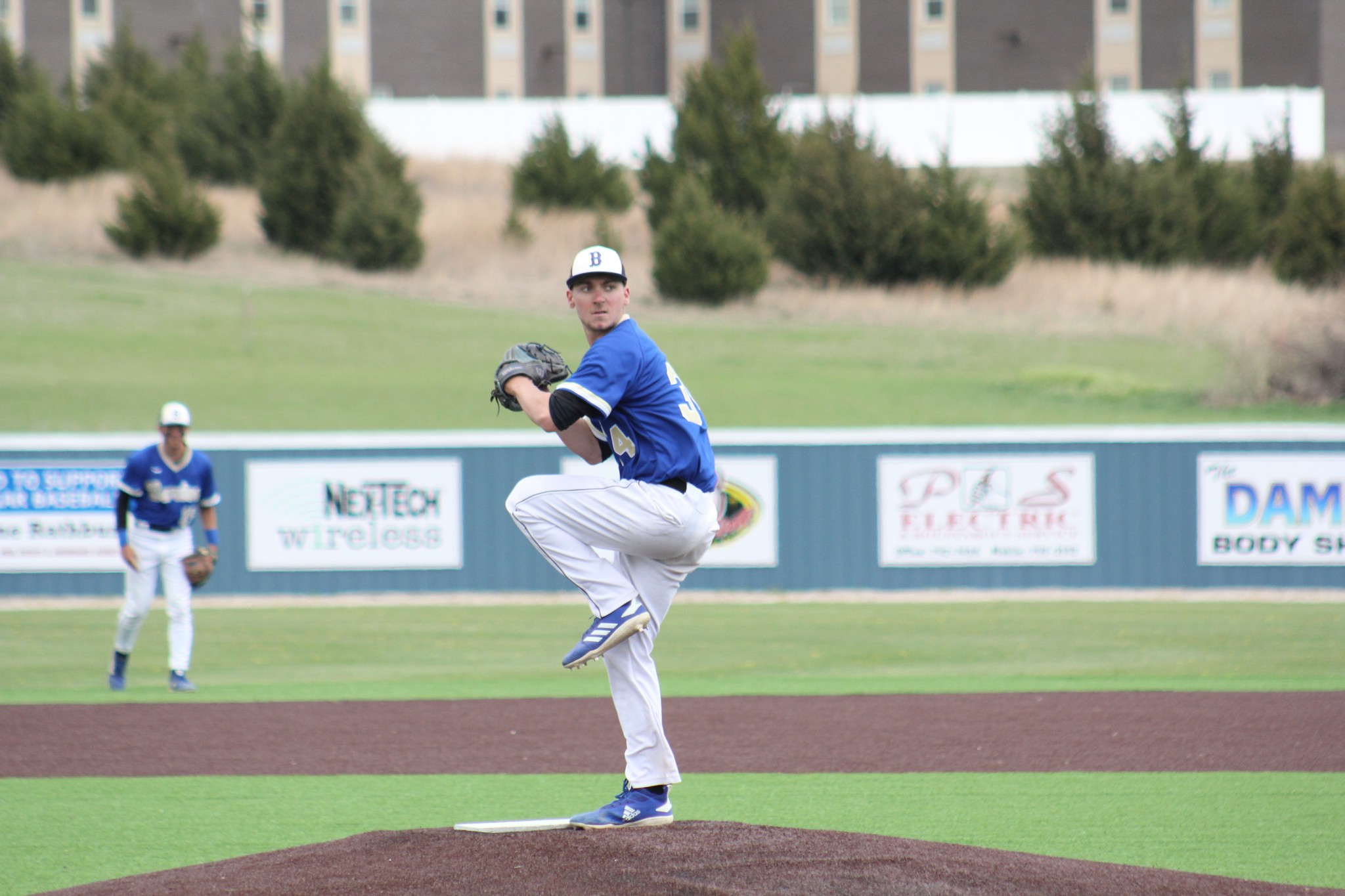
[159,401,191,427]
[566,245,627,291]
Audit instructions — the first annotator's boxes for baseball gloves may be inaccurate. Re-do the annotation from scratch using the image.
[493,341,569,412]
[181,550,217,591]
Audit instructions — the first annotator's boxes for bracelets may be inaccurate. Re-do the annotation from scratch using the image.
[117,529,128,546]
[203,529,220,546]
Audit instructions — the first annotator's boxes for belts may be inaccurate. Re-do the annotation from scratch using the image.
[660,477,687,495]
[149,524,171,532]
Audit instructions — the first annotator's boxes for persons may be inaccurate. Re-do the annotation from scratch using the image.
[503,246,719,828]
[109,402,222,691]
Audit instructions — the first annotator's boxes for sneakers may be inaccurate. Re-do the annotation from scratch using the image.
[562,598,651,670]
[107,660,125,692]
[569,778,674,829]
[168,670,196,691]
